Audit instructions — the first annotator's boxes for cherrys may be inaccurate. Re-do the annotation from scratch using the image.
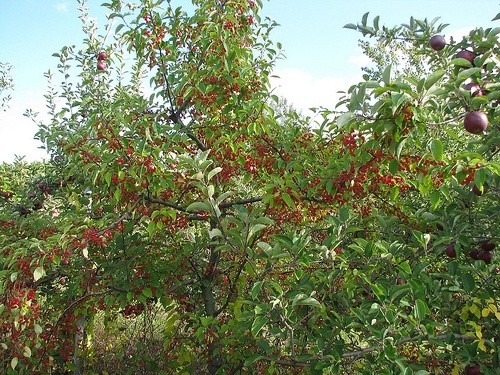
[0,1,487,375]
